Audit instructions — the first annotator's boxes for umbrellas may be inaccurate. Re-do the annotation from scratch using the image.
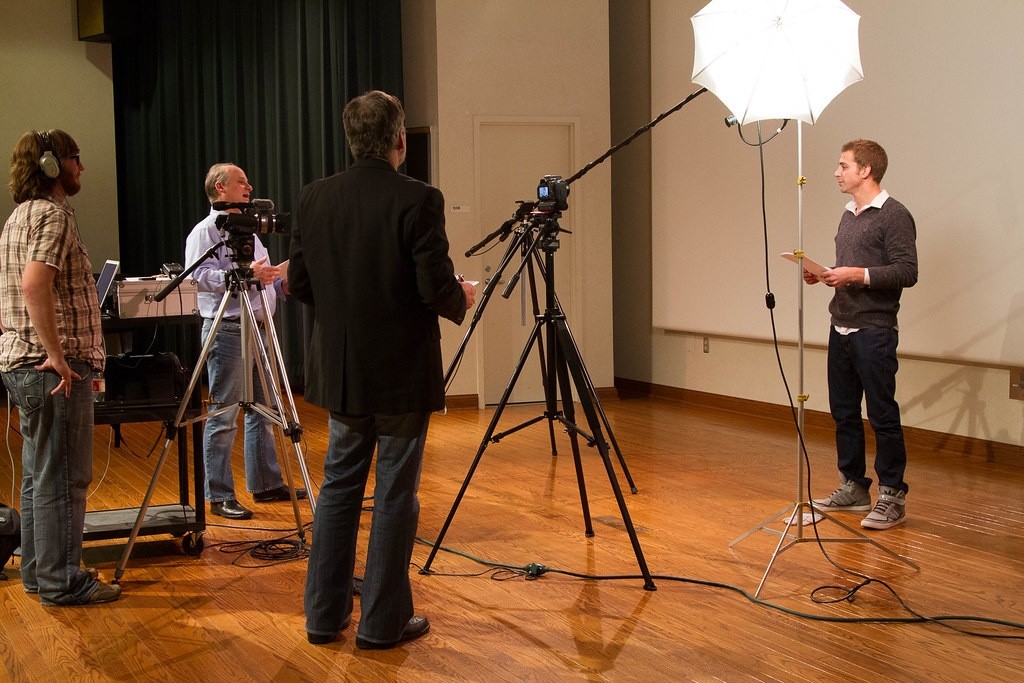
[690,0,865,126]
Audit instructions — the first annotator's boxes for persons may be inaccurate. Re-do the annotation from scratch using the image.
[183,162,309,519]
[0,130,123,609]
[287,91,477,650]
[799,140,918,530]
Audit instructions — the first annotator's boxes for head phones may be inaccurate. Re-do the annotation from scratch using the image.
[36,131,60,178]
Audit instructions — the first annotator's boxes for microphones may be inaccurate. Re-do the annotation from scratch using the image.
[226,198,274,210]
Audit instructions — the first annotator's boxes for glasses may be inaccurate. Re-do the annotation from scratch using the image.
[69,155,81,165]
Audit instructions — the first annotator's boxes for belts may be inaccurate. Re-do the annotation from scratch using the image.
[211,317,265,329]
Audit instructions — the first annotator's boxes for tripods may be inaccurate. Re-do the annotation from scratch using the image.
[114,234,317,580]
[730,119,921,598]
[418,87,708,591]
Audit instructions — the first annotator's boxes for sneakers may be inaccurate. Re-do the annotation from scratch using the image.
[85,582,121,603]
[87,568,98,580]
[861,486,906,528]
[808,471,871,511]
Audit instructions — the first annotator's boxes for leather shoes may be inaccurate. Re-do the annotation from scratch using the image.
[253,484,308,502]
[308,615,352,644]
[356,615,430,649]
[210,499,254,520]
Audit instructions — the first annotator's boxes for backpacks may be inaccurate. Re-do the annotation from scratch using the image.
[0,502,21,580]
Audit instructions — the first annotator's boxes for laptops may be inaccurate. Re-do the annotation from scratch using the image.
[96,260,119,308]
